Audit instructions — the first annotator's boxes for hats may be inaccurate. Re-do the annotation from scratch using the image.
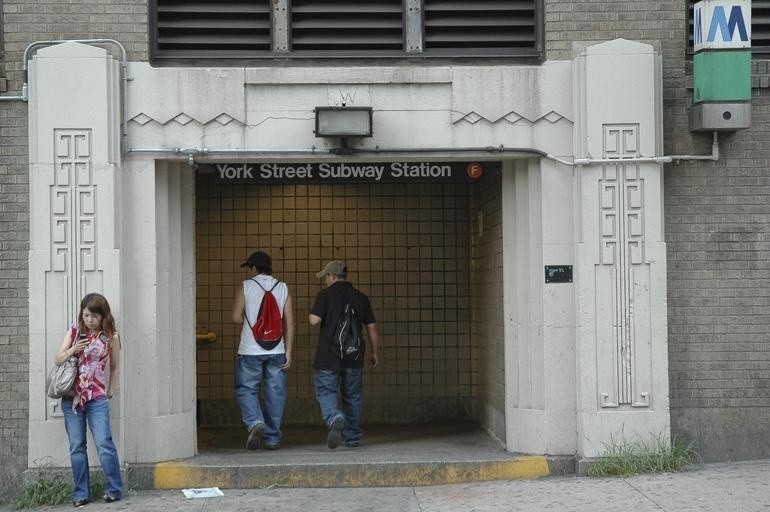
[241,252,271,268]
[316,261,346,278]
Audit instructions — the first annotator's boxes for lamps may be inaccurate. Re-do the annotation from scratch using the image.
[313,106,373,154]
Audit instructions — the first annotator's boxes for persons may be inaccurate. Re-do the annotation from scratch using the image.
[306,261,379,449]
[233,251,295,451]
[53,293,124,507]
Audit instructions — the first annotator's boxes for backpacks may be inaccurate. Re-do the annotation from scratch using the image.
[244,278,284,350]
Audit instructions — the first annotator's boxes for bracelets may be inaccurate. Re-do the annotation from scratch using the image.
[108,391,114,397]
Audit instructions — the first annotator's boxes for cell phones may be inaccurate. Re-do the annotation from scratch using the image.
[80,334,87,339]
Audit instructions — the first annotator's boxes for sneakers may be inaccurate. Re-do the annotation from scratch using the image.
[246,423,279,450]
[103,493,116,502]
[74,500,88,507]
[327,414,359,449]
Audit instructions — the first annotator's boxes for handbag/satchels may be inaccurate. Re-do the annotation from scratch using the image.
[329,314,361,361]
[47,356,78,398]
